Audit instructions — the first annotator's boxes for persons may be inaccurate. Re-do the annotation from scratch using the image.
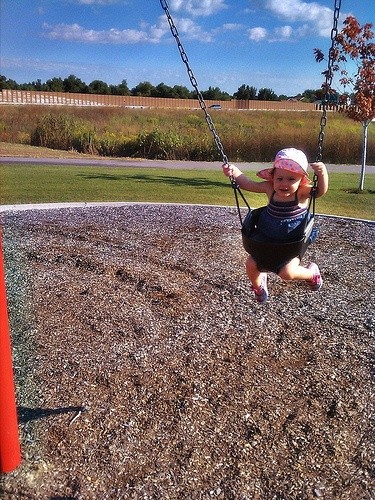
[222,147,329,302]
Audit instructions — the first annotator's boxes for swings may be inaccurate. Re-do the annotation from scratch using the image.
[158,0,343,273]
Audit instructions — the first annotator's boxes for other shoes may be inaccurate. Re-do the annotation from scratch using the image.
[252,272,268,303]
[306,262,323,291]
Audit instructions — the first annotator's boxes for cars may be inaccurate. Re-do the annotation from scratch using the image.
[208,105,222,109]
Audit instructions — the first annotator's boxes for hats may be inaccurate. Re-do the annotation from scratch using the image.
[255,148,312,188]
